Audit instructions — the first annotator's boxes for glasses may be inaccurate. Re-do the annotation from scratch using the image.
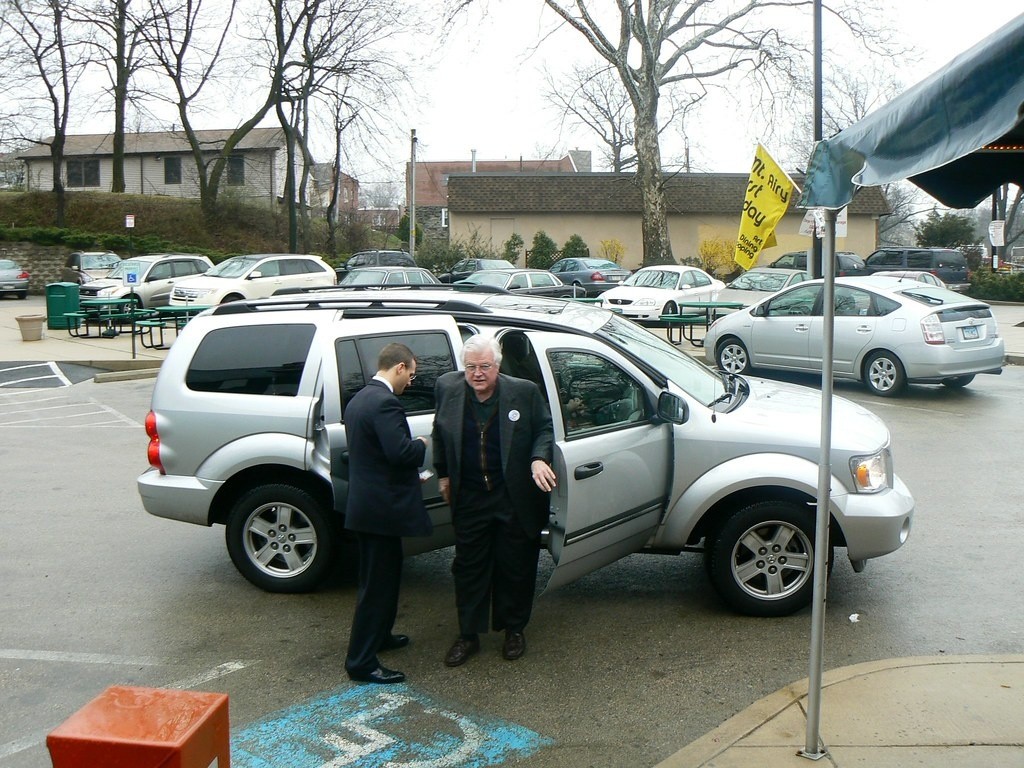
[465,363,494,374]
[395,361,418,380]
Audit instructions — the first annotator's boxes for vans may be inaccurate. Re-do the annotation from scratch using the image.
[768,251,866,276]
[862,248,971,290]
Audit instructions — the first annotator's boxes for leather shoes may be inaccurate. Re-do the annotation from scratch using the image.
[446,634,478,666]
[378,634,408,650]
[351,663,406,683]
[502,626,526,659]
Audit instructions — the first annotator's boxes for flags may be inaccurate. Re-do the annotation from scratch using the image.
[734,141,793,271]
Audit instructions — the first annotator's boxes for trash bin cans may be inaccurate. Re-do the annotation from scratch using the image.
[46,282,81,330]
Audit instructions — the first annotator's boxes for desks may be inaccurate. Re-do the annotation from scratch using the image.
[143,305,210,351]
[568,298,604,307]
[667,302,744,347]
[68,298,139,338]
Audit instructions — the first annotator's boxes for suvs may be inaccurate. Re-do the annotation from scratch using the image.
[137,282,915,618]
[169,254,337,312]
[60,251,124,287]
[315,266,453,293]
[78,253,219,324]
[436,258,516,283]
[334,249,418,285]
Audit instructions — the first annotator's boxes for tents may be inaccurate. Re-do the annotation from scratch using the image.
[802,13,1024,761]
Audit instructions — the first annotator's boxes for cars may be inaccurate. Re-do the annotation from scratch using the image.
[704,276,1004,397]
[594,264,726,321]
[710,268,815,316]
[869,271,946,290]
[453,268,586,298]
[0,260,30,299]
[548,258,633,298]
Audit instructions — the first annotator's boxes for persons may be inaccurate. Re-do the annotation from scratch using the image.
[549,349,585,422]
[431,334,555,669]
[345,342,428,684]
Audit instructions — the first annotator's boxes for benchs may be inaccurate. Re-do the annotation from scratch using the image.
[63,308,195,329]
[658,312,728,327]
